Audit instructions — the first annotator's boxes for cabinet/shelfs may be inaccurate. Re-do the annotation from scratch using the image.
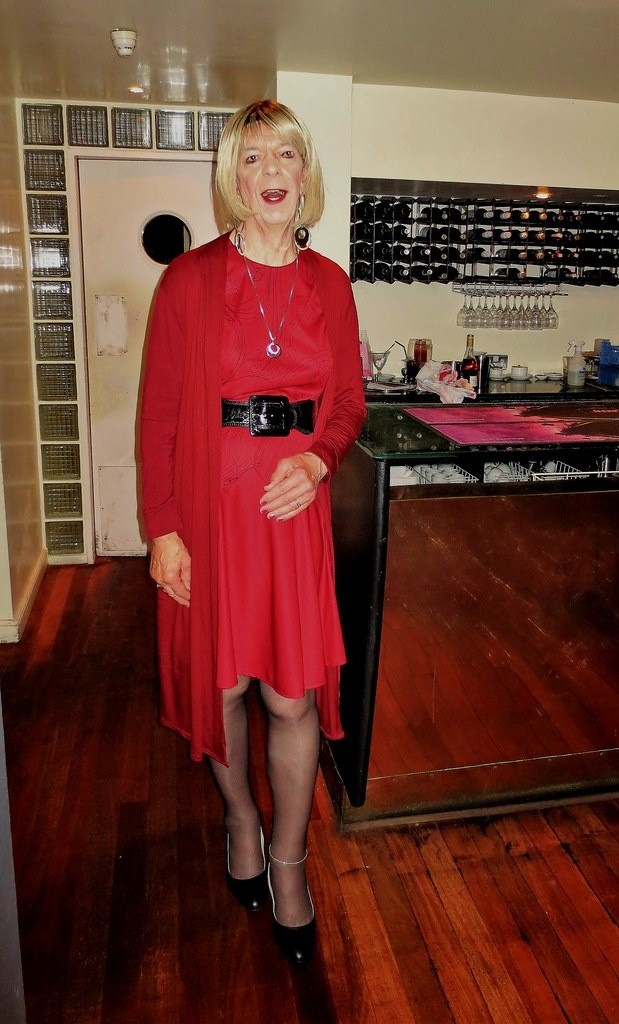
[320,406,619,831]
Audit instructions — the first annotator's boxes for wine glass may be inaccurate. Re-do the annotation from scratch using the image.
[369,351,390,374]
[455,289,559,331]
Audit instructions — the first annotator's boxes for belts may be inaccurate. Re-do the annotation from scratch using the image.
[222,395,314,436]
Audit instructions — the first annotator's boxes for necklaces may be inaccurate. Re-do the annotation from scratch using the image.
[238,221,300,359]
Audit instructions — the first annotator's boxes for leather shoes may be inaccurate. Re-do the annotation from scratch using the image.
[267,862,316,961]
[226,825,268,911]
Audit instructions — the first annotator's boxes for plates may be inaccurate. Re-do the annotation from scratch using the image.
[489,376,506,380]
[510,375,531,380]
[548,376,563,381]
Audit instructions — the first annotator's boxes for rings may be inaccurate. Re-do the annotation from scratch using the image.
[295,500,301,508]
[157,584,163,588]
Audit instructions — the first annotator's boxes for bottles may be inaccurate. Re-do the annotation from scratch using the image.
[407,338,415,360]
[462,334,479,390]
[426,339,433,360]
[414,339,427,362]
[350,202,619,280]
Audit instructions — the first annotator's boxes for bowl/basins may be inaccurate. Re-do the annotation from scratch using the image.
[535,375,548,380]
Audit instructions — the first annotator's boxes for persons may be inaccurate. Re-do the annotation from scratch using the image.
[133,99,367,967]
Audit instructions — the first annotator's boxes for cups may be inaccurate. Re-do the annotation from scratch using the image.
[511,366,528,376]
[490,368,502,376]
[484,463,511,482]
[416,464,466,484]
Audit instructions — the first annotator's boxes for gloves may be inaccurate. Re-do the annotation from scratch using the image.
[415,360,476,404]
